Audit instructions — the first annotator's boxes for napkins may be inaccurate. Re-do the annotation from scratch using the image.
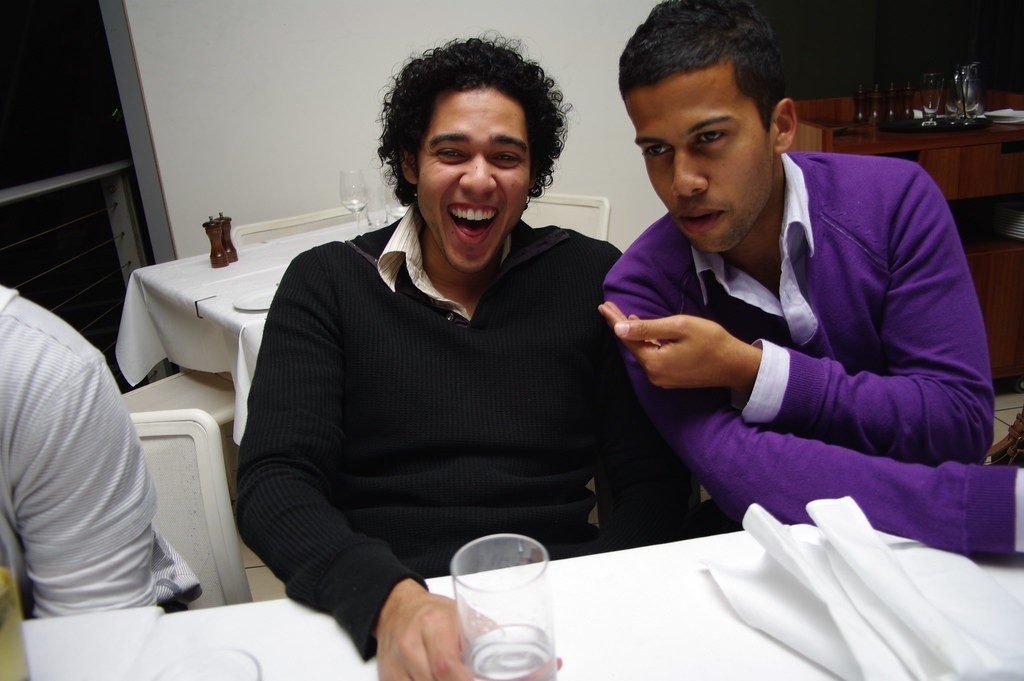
[710,497,1024,681]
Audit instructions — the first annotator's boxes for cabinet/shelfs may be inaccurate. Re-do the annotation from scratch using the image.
[769,90,1024,382]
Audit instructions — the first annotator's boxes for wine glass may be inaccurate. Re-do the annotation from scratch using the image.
[922,73,944,127]
[340,168,369,234]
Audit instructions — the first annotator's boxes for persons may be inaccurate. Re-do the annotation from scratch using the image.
[597,1,1024,555]
[234,30,704,681]
[0,286,204,621]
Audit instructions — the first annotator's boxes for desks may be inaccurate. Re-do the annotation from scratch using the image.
[117,203,404,445]
[0,526,1024,681]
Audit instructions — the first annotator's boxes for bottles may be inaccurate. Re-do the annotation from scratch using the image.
[0,540,30,681]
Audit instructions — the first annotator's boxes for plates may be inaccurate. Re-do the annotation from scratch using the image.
[993,202,1024,242]
[233,286,278,311]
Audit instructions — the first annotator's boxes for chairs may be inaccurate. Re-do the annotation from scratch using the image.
[136,413,246,610]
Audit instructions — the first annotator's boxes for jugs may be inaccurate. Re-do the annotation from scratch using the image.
[947,62,986,117]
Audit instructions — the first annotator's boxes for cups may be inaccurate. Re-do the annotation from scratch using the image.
[449,536,558,681]
[385,187,410,220]
